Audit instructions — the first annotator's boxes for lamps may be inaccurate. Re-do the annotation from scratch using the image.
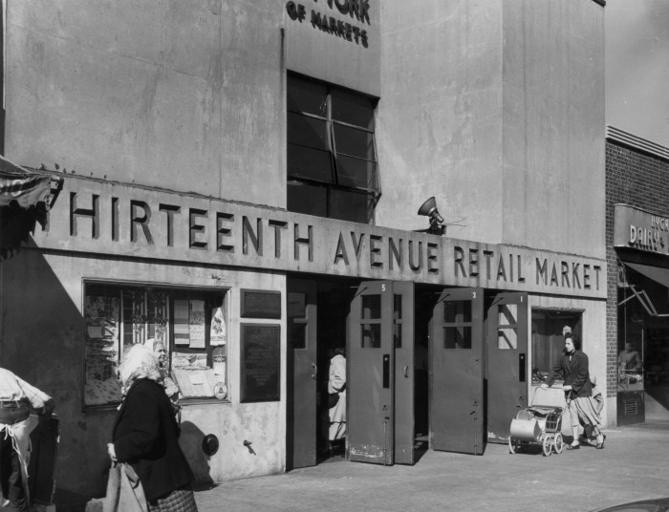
[416,194,446,235]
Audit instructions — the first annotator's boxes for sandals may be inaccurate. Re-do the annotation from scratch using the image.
[566,444,580,449]
[597,433,606,449]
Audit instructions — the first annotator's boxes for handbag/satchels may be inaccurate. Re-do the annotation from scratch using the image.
[102,461,148,512]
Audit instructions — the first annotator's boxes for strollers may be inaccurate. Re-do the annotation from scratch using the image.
[506,383,573,456]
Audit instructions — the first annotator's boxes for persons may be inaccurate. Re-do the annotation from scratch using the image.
[619,340,643,371]
[146,337,181,421]
[106,344,200,512]
[540,333,606,449]
[322,338,347,455]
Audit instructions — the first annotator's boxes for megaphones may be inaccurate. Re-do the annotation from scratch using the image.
[417,196,444,223]
